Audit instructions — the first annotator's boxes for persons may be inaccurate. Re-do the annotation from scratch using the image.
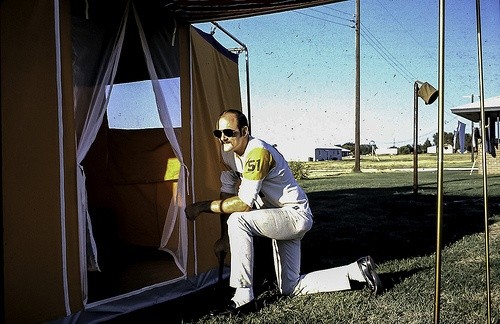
[183,108,381,320]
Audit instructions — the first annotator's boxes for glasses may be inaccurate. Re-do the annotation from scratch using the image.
[213,129,242,137]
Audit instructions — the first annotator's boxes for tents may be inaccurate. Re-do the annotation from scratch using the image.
[0,0,251,324]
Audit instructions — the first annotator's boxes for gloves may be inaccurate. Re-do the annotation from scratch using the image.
[184,201,215,220]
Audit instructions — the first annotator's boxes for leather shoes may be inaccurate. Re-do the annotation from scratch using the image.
[209,299,257,319]
[358,256,380,296]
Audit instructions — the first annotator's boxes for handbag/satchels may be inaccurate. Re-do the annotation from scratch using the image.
[415,80,439,104]
